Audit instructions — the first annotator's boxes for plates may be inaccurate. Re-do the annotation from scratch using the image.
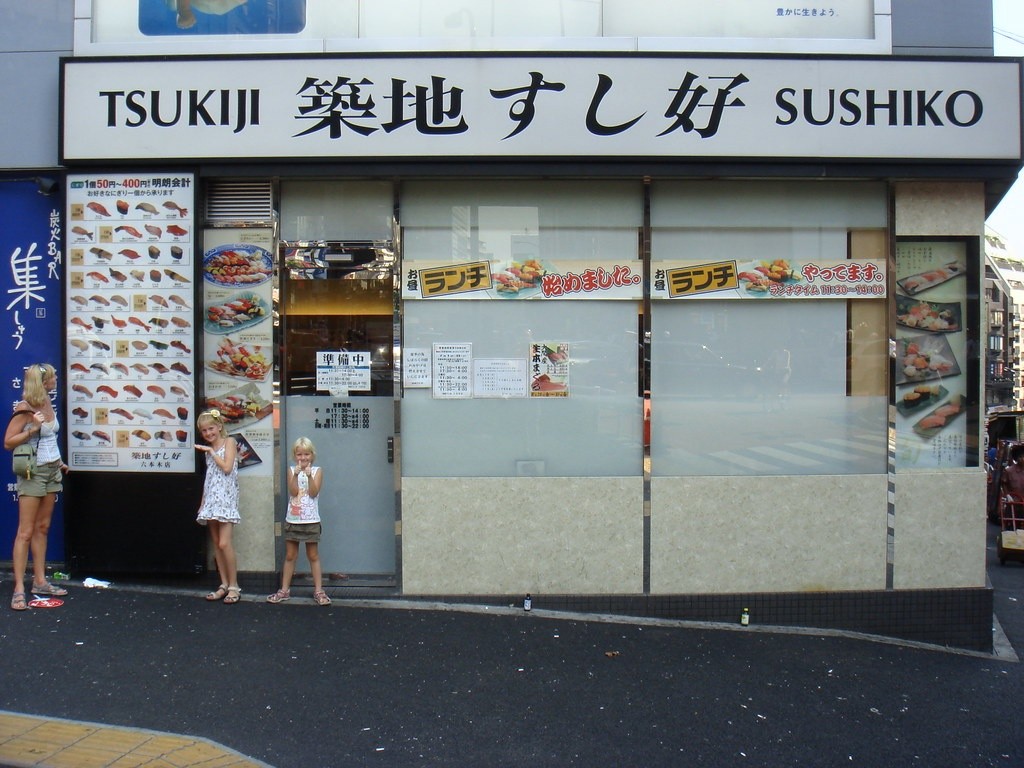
[895,293,962,332]
[203,336,274,383]
[204,243,274,289]
[913,394,966,439]
[204,291,272,334]
[897,261,966,296]
[207,381,272,431]
[486,259,560,299]
[897,385,949,417]
[735,261,798,299]
[896,332,961,385]
[228,433,262,468]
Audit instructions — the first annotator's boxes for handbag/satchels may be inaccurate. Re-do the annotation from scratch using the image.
[12,443,38,480]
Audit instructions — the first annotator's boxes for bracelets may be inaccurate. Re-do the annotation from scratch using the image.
[211,452,215,458]
[28,427,32,439]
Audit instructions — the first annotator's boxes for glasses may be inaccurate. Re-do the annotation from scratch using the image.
[39,362,46,382]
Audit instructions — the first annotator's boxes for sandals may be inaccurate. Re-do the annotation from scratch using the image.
[314,590,332,605]
[31,580,68,596]
[11,592,27,611]
[206,584,228,601]
[224,587,242,604]
[267,588,291,603]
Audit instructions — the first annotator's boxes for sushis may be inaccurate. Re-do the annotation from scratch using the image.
[489,259,555,293]
[208,298,265,328]
[205,392,261,423]
[70,200,191,441]
[738,260,800,291]
[894,261,962,429]
[210,339,269,380]
[209,250,272,283]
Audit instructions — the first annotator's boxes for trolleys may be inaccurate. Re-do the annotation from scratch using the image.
[996,499,1024,566]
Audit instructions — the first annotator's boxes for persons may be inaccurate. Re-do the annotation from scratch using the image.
[4,362,69,610]
[984,440,1024,531]
[266,436,333,606]
[194,408,241,604]
